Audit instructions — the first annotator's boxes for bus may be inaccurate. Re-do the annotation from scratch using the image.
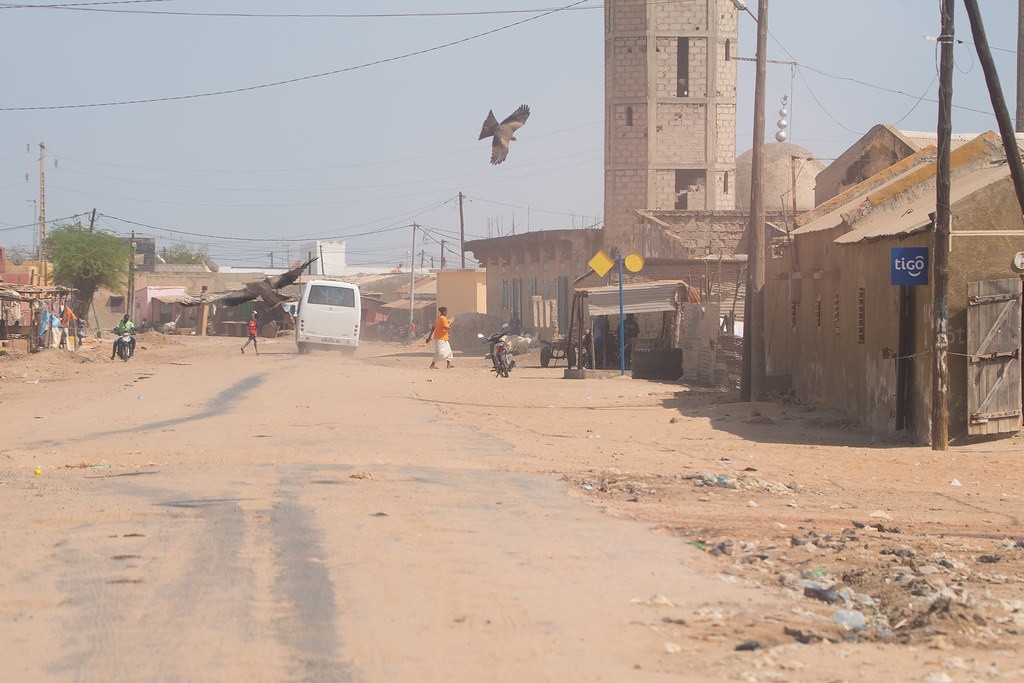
[290,280,362,358]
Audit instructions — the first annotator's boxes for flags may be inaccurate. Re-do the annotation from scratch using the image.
[38,304,77,335]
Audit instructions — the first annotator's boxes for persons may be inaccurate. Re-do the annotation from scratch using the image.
[240,310,260,356]
[142,318,147,328]
[377,319,417,341]
[593,315,610,336]
[426,307,455,369]
[616,313,640,340]
[111,314,136,360]
[510,313,520,336]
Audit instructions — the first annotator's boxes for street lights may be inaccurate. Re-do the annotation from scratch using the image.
[731,0,767,402]
[406,251,410,272]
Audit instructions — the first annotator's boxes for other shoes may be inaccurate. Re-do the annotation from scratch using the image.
[447,365,454,368]
[241,347,245,354]
[111,356,114,360]
[430,365,438,369]
[256,352,260,355]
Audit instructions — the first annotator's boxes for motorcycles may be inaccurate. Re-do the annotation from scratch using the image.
[114,327,137,363]
[478,323,516,377]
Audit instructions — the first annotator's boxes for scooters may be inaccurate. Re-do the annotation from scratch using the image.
[207,324,213,336]
